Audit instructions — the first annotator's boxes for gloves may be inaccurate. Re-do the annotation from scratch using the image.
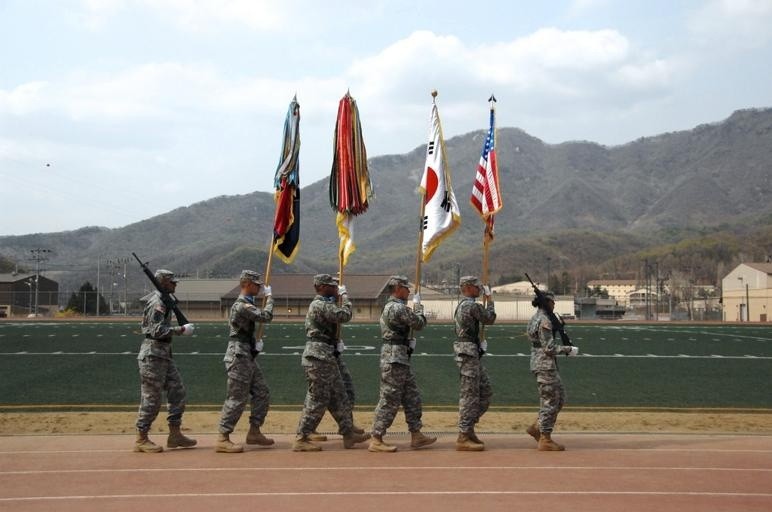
[480,340,488,351]
[337,340,343,351]
[183,323,195,336]
[255,339,264,351]
[481,284,492,297]
[413,293,420,303]
[337,285,347,296]
[263,284,273,297]
[569,346,579,355]
[408,337,417,349]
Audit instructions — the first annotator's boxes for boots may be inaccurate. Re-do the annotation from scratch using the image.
[247,425,273,446]
[134,430,163,452]
[538,430,564,451]
[456,425,484,452]
[167,424,198,448]
[527,420,541,441]
[216,432,243,453]
[368,436,397,452]
[410,431,437,448]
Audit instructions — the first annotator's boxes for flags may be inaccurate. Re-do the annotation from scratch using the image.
[470,108,502,247]
[418,100,461,265]
[272,94,301,262]
[328,91,377,267]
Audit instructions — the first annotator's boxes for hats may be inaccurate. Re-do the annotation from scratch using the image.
[390,276,412,288]
[154,270,179,283]
[240,270,263,284]
[314,275,338,285]
[460,275,482,287]
[536,288,554,299]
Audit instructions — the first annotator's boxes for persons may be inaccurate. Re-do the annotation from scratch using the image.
[369,275,437,453]
[292,275,372,453]
[525,289,579,451]
[216,268,275,452]
[133,269,197,454]
[451,276,497,452]
[307,278,366,442]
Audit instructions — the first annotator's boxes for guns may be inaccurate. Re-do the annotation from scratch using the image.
[524,272,571,345]
[131,252,189,325]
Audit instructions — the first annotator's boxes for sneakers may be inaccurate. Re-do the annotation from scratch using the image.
[307,432,326,441]
[337,425,365,435]
[342,428,371,448]
[291,439,321,452]
